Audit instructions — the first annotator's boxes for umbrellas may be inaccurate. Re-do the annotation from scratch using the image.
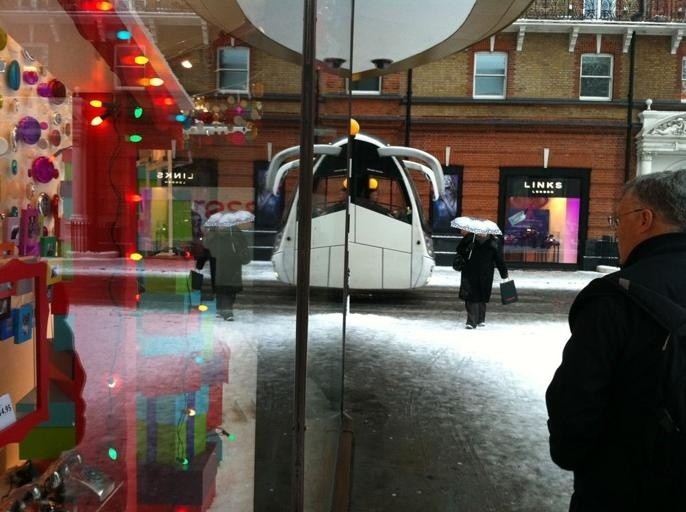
[450,215,504,262]
[201,209,257,255]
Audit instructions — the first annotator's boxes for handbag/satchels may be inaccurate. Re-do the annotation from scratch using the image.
[500,280,519,305]
[187,270,203,290]
[453,253,472,271]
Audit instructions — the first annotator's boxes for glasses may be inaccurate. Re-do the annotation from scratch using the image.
[608,208,643,230]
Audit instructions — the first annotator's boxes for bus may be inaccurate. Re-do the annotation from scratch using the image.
[348,133,447,289]
[266,140,348,286]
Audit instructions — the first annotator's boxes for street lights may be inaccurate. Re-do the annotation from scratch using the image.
[349,119,360,180]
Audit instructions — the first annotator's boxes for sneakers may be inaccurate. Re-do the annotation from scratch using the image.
[465,322,486,329]
[216,311,234,321]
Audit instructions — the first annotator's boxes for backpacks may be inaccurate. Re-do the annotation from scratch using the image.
[602,273,686,490]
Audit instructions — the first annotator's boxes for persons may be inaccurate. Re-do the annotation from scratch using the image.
[544,167,686,510]
[453,234,508,330]
[194,225,254,327]
